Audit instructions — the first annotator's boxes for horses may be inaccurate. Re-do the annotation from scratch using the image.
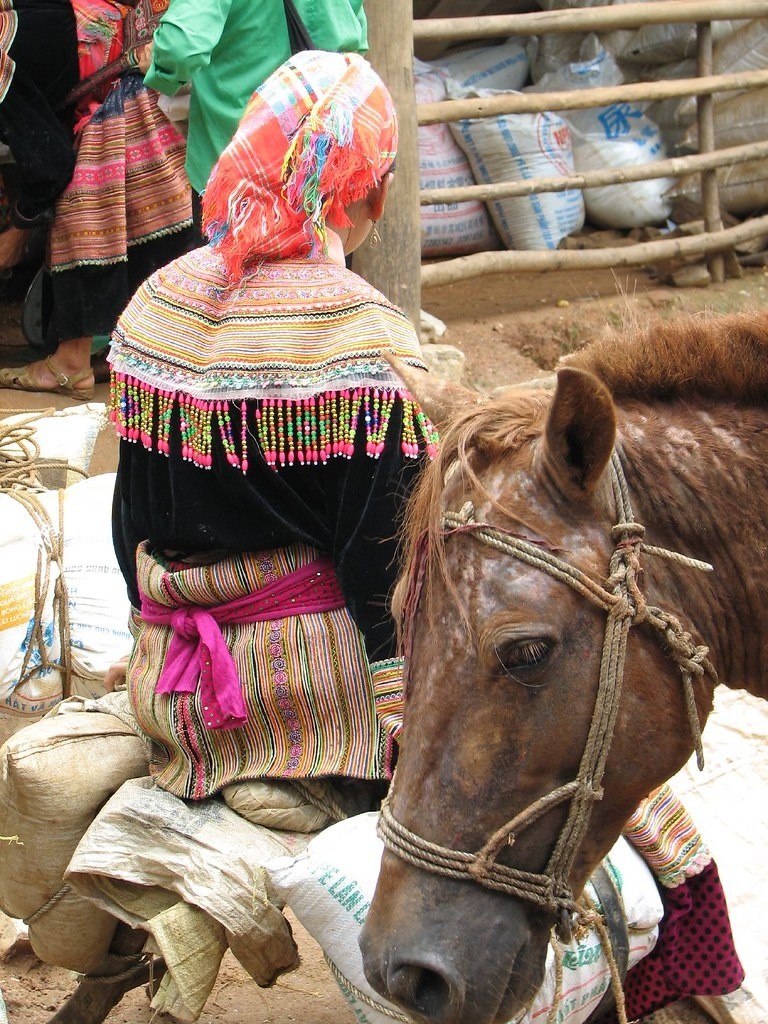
[355,266,768,1024]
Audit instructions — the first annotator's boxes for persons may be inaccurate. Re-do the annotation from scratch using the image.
[106,47,746,1024]
[0,0,371,400]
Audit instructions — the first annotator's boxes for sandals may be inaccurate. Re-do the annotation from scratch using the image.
[0,354,95,402]
